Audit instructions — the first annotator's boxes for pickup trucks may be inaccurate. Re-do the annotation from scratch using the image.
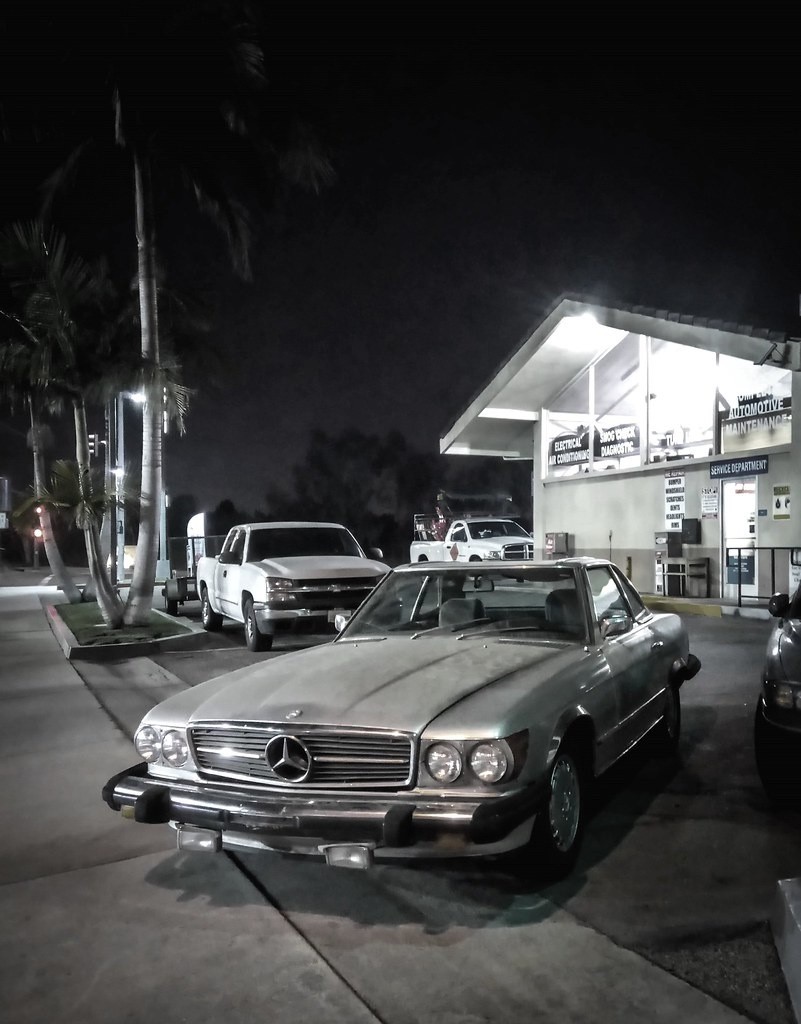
[196,521,403,650]
[410,518,535,564]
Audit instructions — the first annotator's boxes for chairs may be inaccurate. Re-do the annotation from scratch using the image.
[426,587,593,648]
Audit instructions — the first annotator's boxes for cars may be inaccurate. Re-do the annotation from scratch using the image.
[753,546,801,809]
[99,558,702,881]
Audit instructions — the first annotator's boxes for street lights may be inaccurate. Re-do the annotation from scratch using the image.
[117,392,147,579]
